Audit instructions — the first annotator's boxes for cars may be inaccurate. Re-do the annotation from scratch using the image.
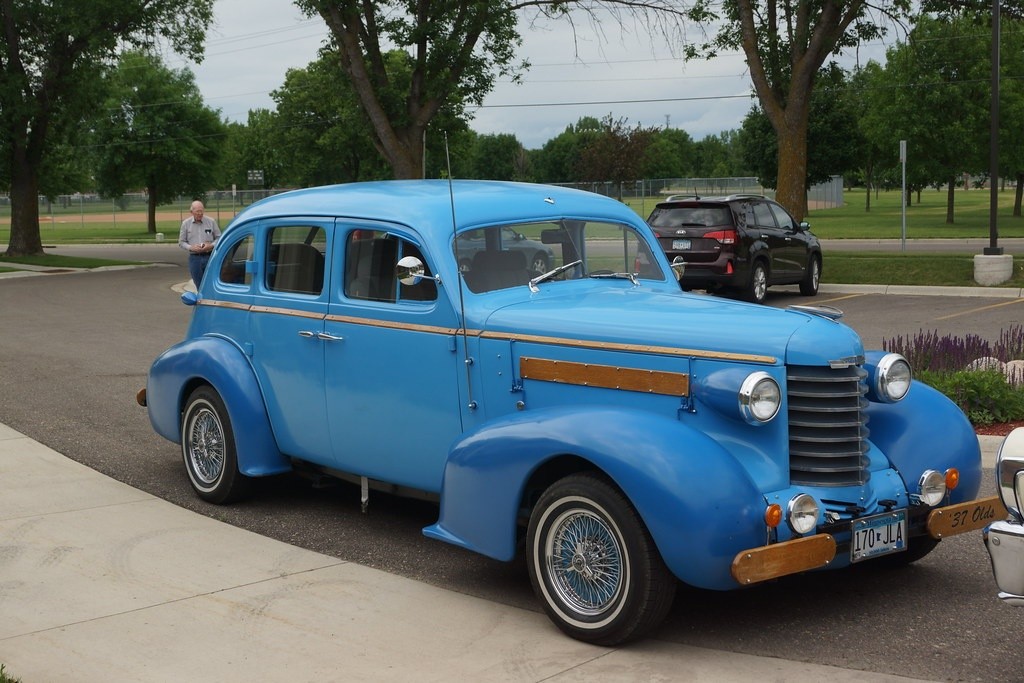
[135,179,1024,649]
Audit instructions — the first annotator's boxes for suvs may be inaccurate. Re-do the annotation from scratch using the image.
[633,183,824,303]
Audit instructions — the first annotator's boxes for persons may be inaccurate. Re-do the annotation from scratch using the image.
[178,201,222,292]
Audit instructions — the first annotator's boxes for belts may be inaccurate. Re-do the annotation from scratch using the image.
[190,253,211,257]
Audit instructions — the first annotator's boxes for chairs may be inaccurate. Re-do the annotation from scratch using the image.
[268,243,325,295]
[348,238,430,301]
[473,250,523,292]
